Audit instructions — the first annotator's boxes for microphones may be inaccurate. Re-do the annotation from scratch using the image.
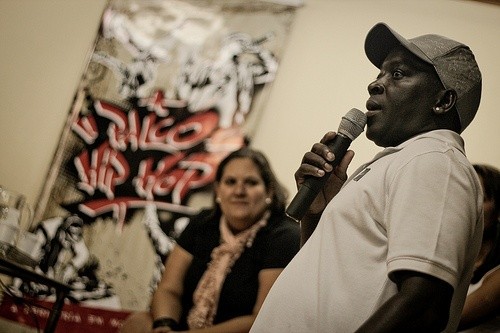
[285,107,368,223]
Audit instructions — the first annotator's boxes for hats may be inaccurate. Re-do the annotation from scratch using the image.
[363,21,482,134]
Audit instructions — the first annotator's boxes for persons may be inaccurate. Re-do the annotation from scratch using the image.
[455,163,500,333]
[117,148,303,333]
[245,20,486,333]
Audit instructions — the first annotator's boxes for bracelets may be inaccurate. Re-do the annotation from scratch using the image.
[151,316,180,332]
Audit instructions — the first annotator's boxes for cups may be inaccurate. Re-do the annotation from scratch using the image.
[0,219,18,243]
